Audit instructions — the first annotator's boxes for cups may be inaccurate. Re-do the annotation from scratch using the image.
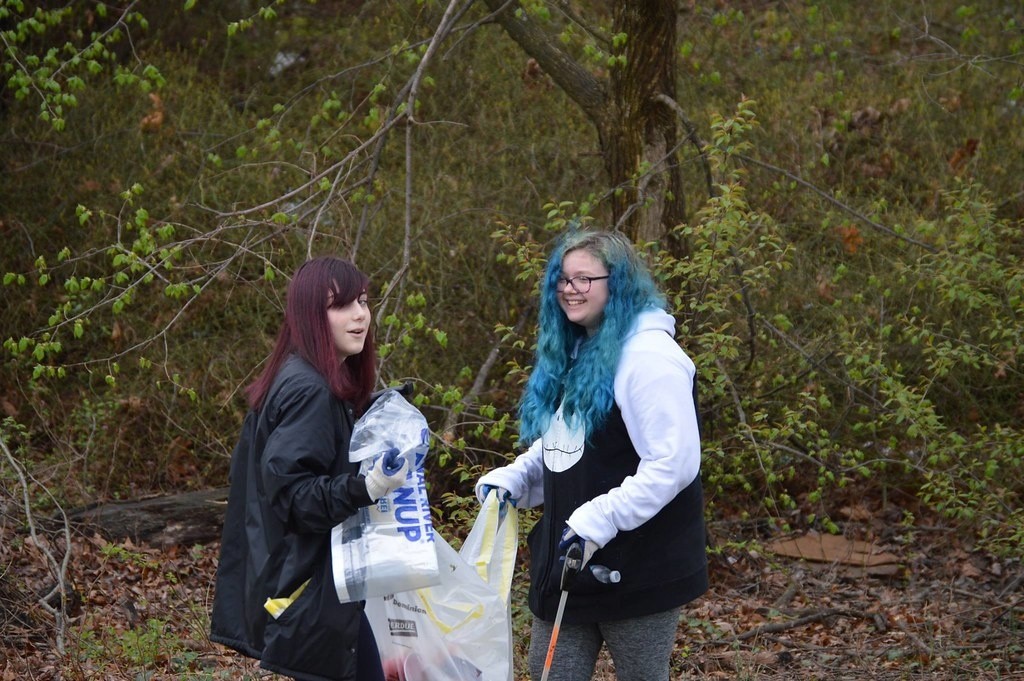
[382,653,424,681]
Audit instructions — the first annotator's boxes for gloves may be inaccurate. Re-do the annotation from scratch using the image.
[364,447,409,503]
[478,484,517,518]
[558,526,599,578]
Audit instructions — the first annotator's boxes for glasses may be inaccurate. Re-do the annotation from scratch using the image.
[555,275,609,293]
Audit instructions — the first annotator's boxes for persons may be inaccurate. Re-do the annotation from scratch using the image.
[209,257,409,681]
[475,226,708,681]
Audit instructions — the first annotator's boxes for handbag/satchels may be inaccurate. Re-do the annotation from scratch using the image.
[364,487,517,681]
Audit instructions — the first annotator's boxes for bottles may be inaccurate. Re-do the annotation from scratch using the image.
[589,565,621,584]
